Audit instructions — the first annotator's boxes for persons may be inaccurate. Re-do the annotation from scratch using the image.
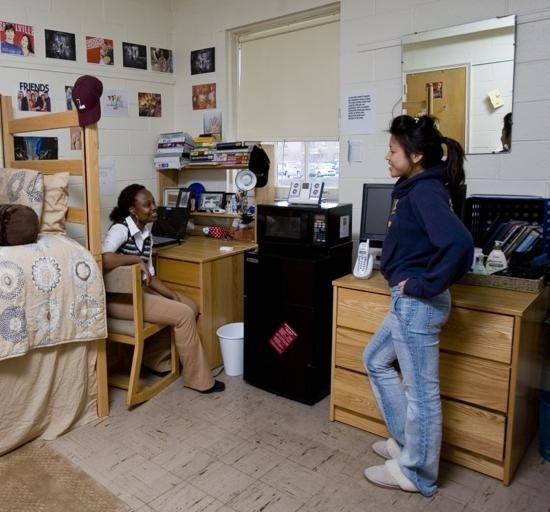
[362,115,476,499]
[100,41,108,57]
[501,112,511,152]
[66,87,72,110]
[0,23,24,55]
[41,94,48,111]
[22,89,31,111]
[29,93,36,110]
[34,90,44,111]
[18,91,24,109]
[44,92,50,112]
[101,183,225,394]
[20,33,34,55]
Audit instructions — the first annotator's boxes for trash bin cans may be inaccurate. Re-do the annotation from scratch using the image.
[216,322,244,376]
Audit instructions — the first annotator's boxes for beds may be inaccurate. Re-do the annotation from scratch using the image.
[0,96,110,454]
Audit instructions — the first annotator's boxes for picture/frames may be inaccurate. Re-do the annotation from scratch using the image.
[176,188,190,208]
[162,187,179,209]
[199,191,226,212]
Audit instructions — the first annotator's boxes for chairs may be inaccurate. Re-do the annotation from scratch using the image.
[104,264,181,409]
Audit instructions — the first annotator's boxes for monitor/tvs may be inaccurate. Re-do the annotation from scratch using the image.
[359,183,467,241]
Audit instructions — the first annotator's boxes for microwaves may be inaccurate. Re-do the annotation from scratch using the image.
[256,203,354,249]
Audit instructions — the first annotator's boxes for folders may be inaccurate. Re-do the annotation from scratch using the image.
[153,131,195,168]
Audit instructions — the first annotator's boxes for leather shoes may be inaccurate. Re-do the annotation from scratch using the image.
[212,380,225,391]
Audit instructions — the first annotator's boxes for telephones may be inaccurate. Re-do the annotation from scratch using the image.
[353,239,374,279]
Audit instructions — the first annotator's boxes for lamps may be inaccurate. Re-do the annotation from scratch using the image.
[235,168,257,224]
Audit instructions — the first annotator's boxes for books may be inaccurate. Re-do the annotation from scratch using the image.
[189,133,219,166]
[490,220,543,260]
[154,131,193,170]
[215,141,261,167]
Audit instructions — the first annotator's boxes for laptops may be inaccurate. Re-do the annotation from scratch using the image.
[150,206,188,248]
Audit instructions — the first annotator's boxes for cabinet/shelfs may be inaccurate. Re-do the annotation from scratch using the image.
[327,268,549,487]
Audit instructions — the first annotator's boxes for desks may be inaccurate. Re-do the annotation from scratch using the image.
[146,232,255,372]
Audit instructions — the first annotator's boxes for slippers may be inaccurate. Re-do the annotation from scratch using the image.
[363,458,419,492]
[371,438,402,459]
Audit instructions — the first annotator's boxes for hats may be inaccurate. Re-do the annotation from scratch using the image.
[71,75,103,127]
[248,144,270,188]
